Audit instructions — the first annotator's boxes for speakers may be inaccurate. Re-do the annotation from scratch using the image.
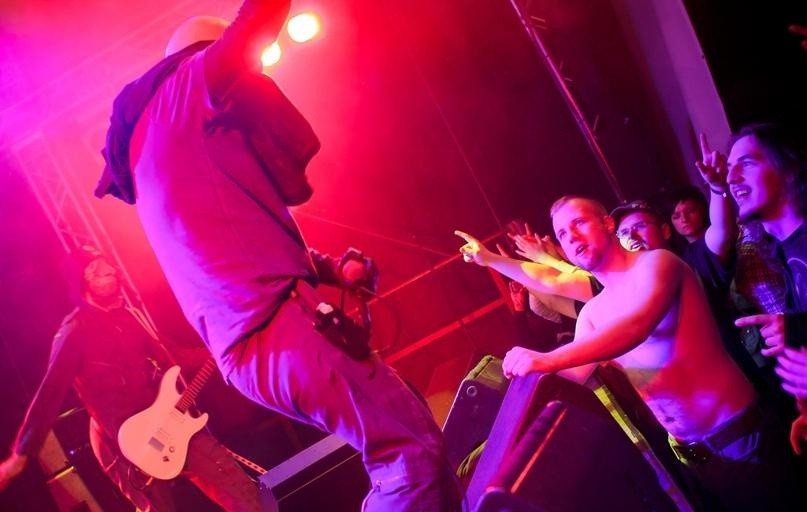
[0,407,134,511]
[442,355,511,480]
[461,369,683,511]
[256,432,372,512]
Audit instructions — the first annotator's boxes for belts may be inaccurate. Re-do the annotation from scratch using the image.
[671,406,760,464]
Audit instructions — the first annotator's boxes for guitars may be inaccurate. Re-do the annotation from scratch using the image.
[118,358,216,480]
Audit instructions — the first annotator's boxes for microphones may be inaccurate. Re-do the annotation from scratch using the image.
[342,260,376,299]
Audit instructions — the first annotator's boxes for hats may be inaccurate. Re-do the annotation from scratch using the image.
[609,199,653,218]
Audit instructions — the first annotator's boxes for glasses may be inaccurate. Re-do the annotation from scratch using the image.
[617,220,656,239]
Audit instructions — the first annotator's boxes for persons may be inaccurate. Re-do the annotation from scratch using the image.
[0,244,278,511]
[125,3,464,511]
